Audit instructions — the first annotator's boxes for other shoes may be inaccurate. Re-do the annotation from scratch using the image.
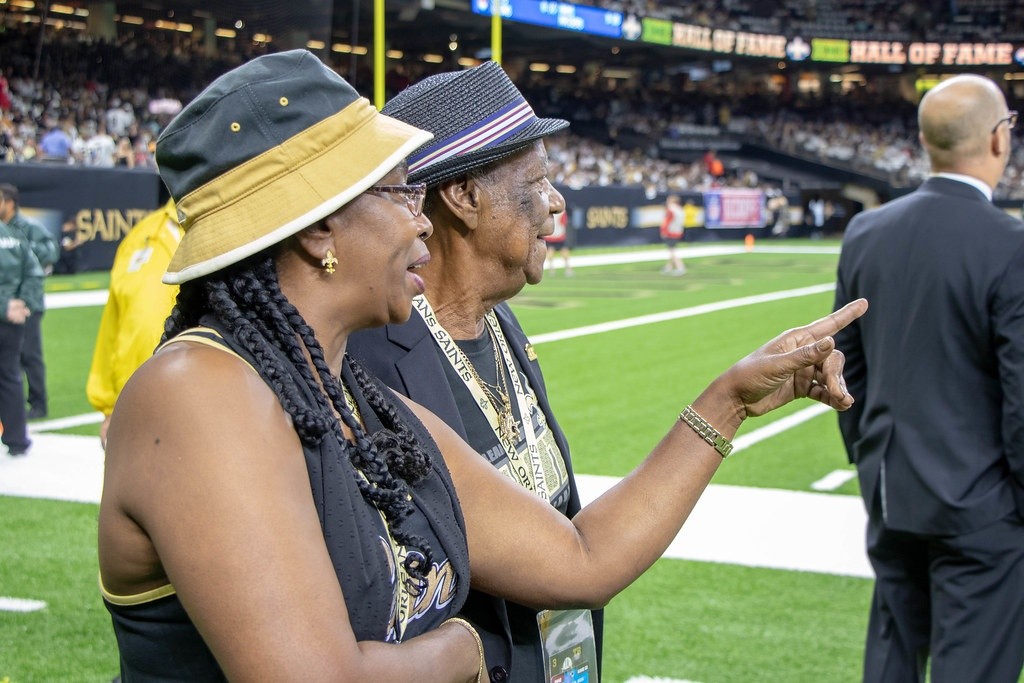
[7,450,26,456]
[28,407,49,419]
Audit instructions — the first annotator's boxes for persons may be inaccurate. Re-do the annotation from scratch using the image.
[98,49,867,683]
[0,0,1024,275]
[0,183,59,456]
[349,62,606,682]
[831,76,1023,682]
[86,197,184,450]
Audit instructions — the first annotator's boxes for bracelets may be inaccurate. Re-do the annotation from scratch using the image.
[678,405,734,458]
[438,618,484,683]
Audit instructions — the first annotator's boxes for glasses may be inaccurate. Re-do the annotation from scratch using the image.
[993,110,1019,133]
[367,181,427,217]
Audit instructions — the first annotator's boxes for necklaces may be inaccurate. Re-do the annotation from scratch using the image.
[452,320,523,446]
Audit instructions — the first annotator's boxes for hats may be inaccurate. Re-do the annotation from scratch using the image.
[160,48,434,288]
[376,61,570,190]
